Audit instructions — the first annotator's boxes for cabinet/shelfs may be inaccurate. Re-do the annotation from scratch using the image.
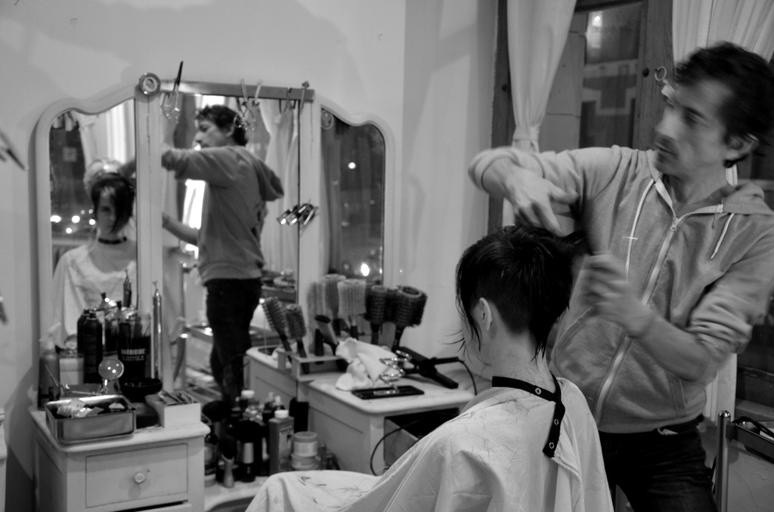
[204,474,271,512]
[246,345,297,408]
[177,317,279,397]
[28,407,211,512]
[308,374,474,474]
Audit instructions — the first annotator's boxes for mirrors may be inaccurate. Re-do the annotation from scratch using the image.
[35,81,394,399]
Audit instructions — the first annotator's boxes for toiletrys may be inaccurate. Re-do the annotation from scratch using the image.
[238,395,293,484]
[117,316,149,404]
[96,292,132,356]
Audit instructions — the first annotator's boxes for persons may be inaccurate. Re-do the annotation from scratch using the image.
[159,105,285,403]
[244,220,618,512]
[52,173,138,351]
[466,38,773,511]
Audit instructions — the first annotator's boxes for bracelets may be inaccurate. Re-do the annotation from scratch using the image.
[624,302,659,339]
